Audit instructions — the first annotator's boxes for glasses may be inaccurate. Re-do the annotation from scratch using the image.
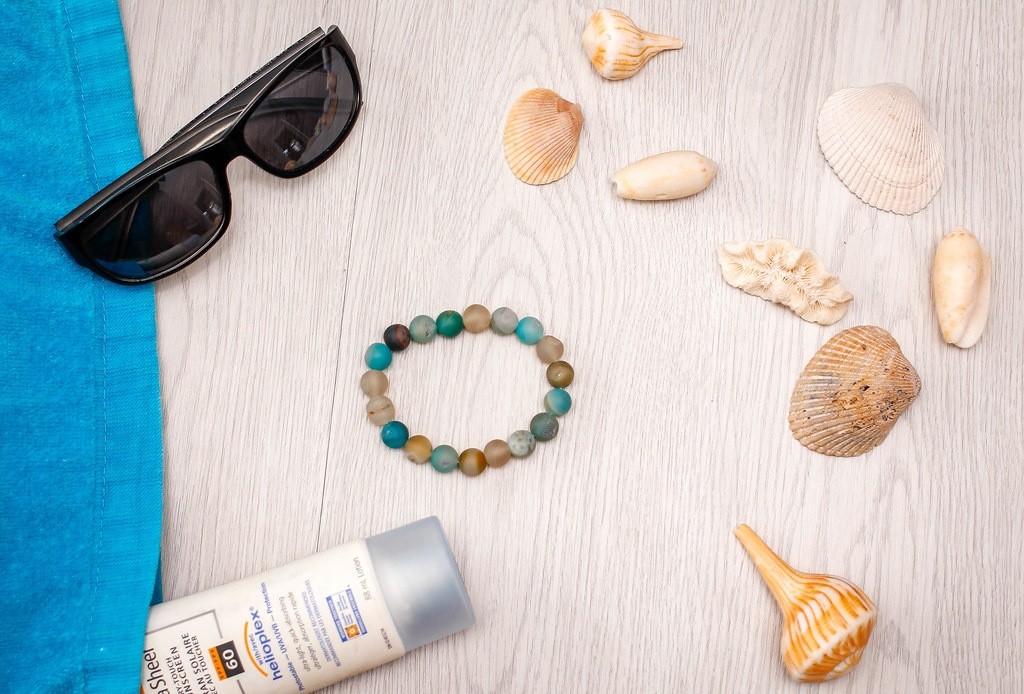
[53,24,363,286]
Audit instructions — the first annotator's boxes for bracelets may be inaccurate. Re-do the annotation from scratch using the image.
[360,305,574,478]
